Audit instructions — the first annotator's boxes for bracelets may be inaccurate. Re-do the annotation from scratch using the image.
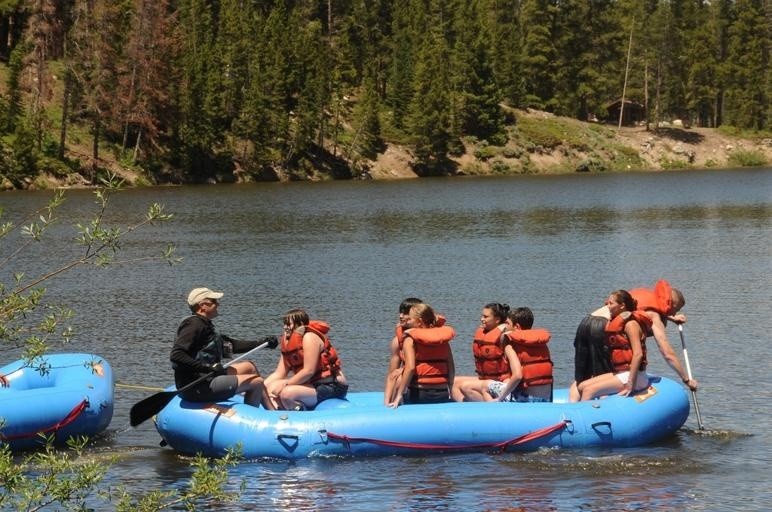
[682,378,690,383]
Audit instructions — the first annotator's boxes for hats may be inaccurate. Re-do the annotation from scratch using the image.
[188,288,224,307]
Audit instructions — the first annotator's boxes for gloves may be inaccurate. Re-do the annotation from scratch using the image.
[208,364,225,374]
[262,336,278,349]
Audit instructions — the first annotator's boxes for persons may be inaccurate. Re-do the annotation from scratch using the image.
[264,309,349,409]
[569,279,698,403]
[479,306,552,402]
[449,302,514,403]
[0,374,10,388]
[169,287,276,410]
[577,289,649,401]
[387,303,456,408]
[384,297,423,405]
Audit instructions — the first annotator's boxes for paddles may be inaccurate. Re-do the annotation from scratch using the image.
[129,339,268,427]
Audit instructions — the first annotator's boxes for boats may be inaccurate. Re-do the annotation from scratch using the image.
[1,352,116,451]
[152,370,691,461]
